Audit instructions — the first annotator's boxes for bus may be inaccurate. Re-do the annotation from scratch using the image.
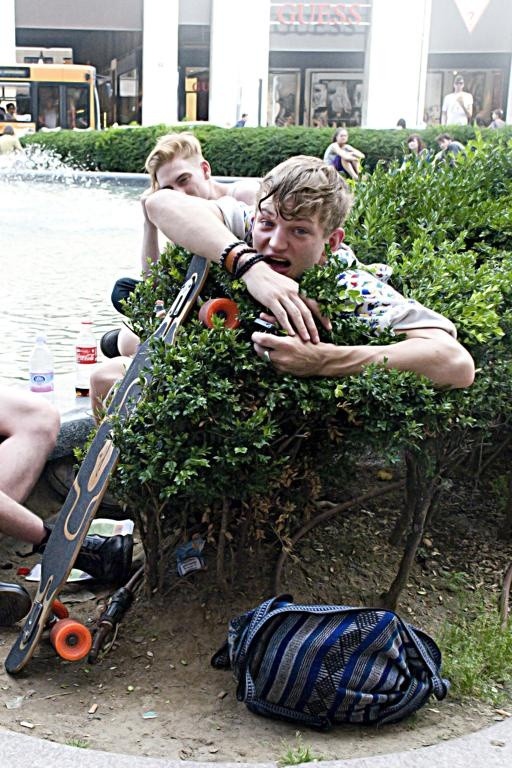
[0,61,114,141]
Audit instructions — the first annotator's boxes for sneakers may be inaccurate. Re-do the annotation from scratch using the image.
[46,466,123,510]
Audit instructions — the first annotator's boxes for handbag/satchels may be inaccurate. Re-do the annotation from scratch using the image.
[211,593,450,730]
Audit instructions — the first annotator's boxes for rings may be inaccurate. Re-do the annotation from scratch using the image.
[264,348,273,361]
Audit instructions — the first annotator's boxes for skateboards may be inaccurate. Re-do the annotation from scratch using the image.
[5,255,240,675]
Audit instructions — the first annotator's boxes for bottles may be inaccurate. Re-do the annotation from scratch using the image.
[29,330,55,399]
[74,318,99,398]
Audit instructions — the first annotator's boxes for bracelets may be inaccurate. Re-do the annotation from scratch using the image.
[219,239,264,283]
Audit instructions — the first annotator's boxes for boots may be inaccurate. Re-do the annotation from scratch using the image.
[0,522,134,629]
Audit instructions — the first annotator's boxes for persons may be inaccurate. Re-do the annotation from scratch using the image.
[396,119,406,129]
[0,387,134,626]
[120,108,130,124]
[238,114,247,128]
[1,87,77,157]
[102,132,263,357]
[91,154,476,427]
[271,83,362,128]
[417,76,507,129]
[323,126,468,182]
